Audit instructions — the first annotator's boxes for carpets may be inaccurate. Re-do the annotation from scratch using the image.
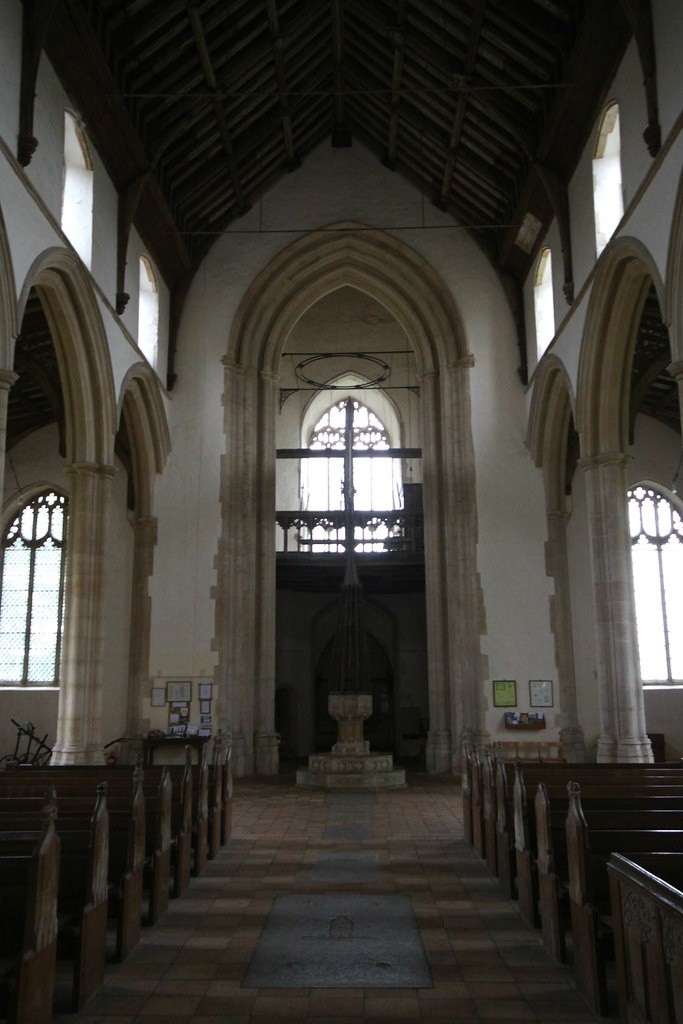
[240,894,436,990]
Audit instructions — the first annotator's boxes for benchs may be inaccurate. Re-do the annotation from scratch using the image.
[0,759,235,1024]
[460,739,683,1024]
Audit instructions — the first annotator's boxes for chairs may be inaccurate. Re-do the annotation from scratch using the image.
[493,740,568,765]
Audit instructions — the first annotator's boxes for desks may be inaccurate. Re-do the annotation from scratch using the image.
[142,736,204,766]
